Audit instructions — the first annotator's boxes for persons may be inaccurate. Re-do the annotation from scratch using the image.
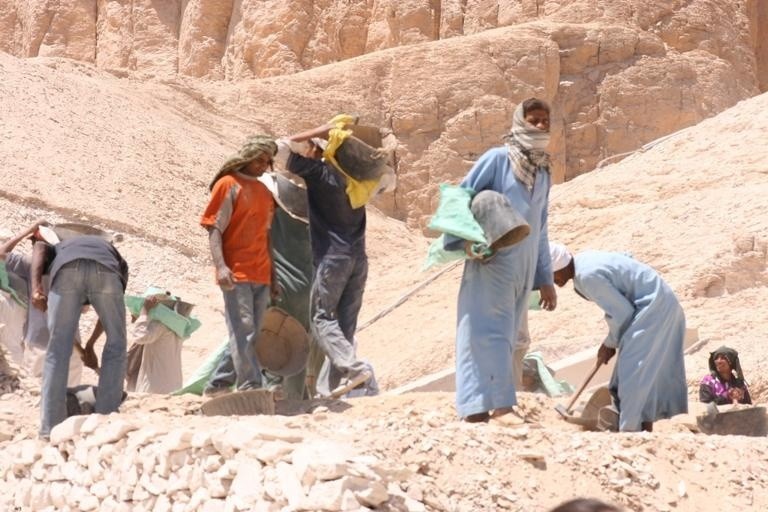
[257,137,325,397]
[201,134,279,394]
[277,114,398,397]
[547,239,689,431]
[0,219,89,389]
[128,284,184,396]
[551,498,619,512]
[30,225,128,441]
[442,98,557,425]
[699,346,752,405]
[523,351,578,395]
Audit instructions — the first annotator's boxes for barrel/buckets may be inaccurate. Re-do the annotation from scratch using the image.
[581,384,652,432]
[258,304,310,382]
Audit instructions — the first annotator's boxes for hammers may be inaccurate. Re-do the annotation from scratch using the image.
[74,337,128,407]
[554,361,603,419]
